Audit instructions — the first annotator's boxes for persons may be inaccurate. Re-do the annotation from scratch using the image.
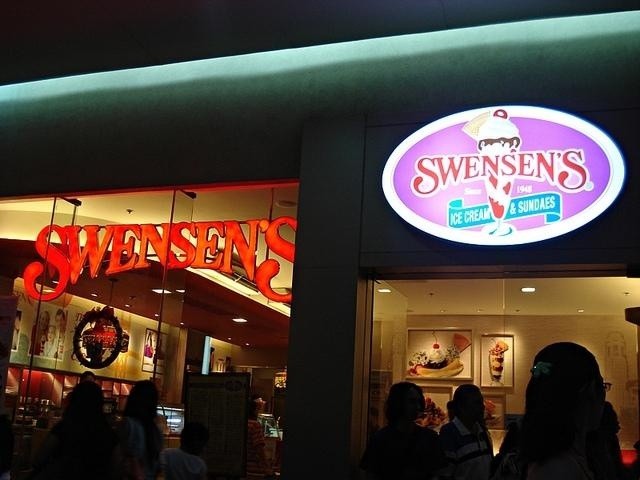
[29,307,65,360]
[144,330,163,360]
[355,342,621,479]
[34,371,209,480]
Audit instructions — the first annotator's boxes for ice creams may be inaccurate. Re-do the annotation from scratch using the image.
[476,109,522,217]
[489,337,509,380]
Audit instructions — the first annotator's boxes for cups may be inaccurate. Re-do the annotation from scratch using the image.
[488,353,505,386]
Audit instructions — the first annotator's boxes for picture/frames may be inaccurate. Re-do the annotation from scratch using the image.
[140,327,168,376]
[10,301,70,363]
[404,326,515,429]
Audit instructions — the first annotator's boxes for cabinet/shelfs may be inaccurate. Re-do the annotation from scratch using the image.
[50,374,132,416]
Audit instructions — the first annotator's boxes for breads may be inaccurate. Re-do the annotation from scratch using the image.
[417,360,464,378]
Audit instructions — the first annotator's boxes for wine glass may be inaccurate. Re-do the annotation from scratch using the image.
[481,176,515,236]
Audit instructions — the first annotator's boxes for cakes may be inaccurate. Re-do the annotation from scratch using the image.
[426,330,448,369]
